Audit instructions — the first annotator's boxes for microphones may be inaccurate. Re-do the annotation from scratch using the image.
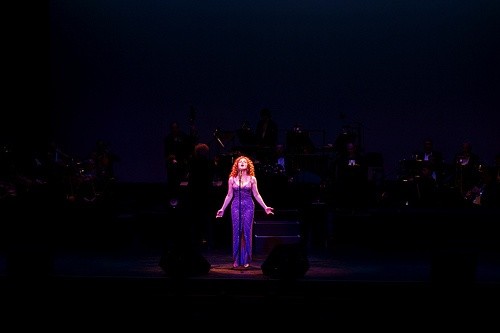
[239,168,241,176]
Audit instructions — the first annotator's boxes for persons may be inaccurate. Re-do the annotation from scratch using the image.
[338,143,363,210]
[215,155,276,270]
[415,141,475,200]
[171,144,216,277]
[2,138,116,201]
[163,109,318,188]
